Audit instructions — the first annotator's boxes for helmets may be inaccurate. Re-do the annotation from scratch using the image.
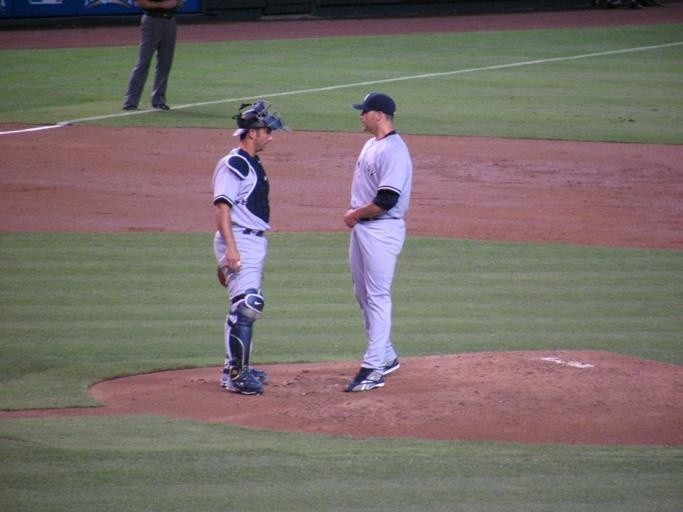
[232,99,289,137]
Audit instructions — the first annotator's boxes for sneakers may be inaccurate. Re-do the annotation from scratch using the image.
[225,371,264,395]
[220,367,267,387]
[344,367,385,391]
[153,104,170,110]
[382,357,400,375]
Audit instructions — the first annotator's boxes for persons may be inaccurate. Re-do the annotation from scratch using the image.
[213,102,290,398]
[339,94,413,393]
[120,0,181,112]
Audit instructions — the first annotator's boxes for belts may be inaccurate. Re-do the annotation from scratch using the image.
[243,227,264,236]
[361,216,399,220]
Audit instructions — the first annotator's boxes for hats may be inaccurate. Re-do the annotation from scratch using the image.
[352,91,396,114]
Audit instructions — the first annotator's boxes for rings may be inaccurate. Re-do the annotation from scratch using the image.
[236,260,242,268]
